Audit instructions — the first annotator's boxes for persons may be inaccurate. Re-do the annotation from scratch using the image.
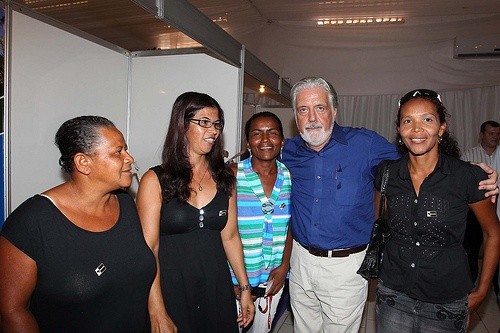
[460,121,500,298]
[373,89,500,333]
[136,91,255,333]
[277,77,499,333]
[226,111,293,333]
[0,115,157,333]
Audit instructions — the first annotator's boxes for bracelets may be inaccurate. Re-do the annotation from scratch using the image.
[238,285,253,293]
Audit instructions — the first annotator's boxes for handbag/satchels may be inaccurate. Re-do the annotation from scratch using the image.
[357,160,391,280]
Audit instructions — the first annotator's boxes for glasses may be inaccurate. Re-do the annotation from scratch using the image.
[188,118,223,130]
[398,89,442,109]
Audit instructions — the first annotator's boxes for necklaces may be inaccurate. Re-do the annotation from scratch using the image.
[193,167,209,191]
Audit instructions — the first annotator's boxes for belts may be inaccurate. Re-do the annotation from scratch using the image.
[291,233,367,257]
